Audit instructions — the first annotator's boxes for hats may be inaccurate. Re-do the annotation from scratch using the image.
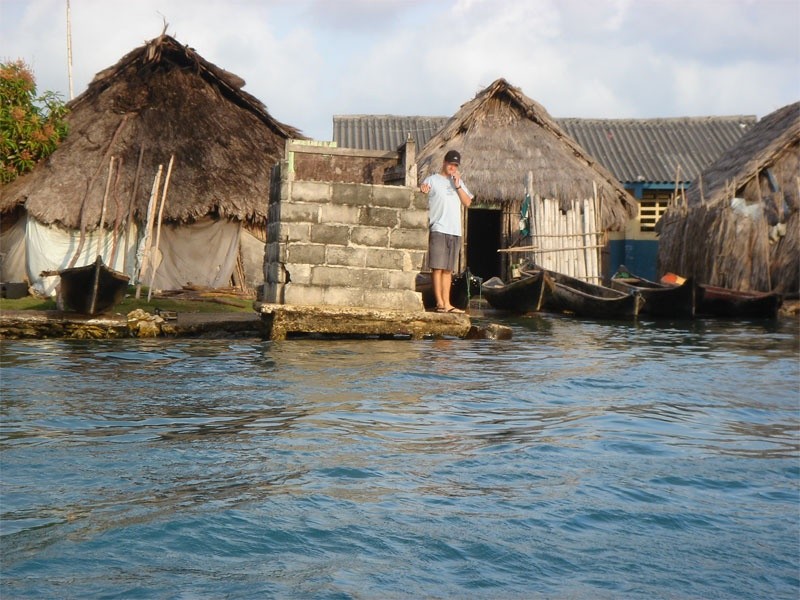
[445,150,460,165]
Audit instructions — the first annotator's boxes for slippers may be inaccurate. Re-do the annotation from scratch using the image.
[434,306,466,314]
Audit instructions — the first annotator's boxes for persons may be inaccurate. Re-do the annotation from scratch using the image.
[421,150,474,313]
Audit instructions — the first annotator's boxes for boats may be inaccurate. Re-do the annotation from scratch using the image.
[481,269,557,311]
[659,269,783,320]
[519,265,645,317]
[611,264,705,317]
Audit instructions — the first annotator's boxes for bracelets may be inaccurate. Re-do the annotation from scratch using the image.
[456,185,461,189]
